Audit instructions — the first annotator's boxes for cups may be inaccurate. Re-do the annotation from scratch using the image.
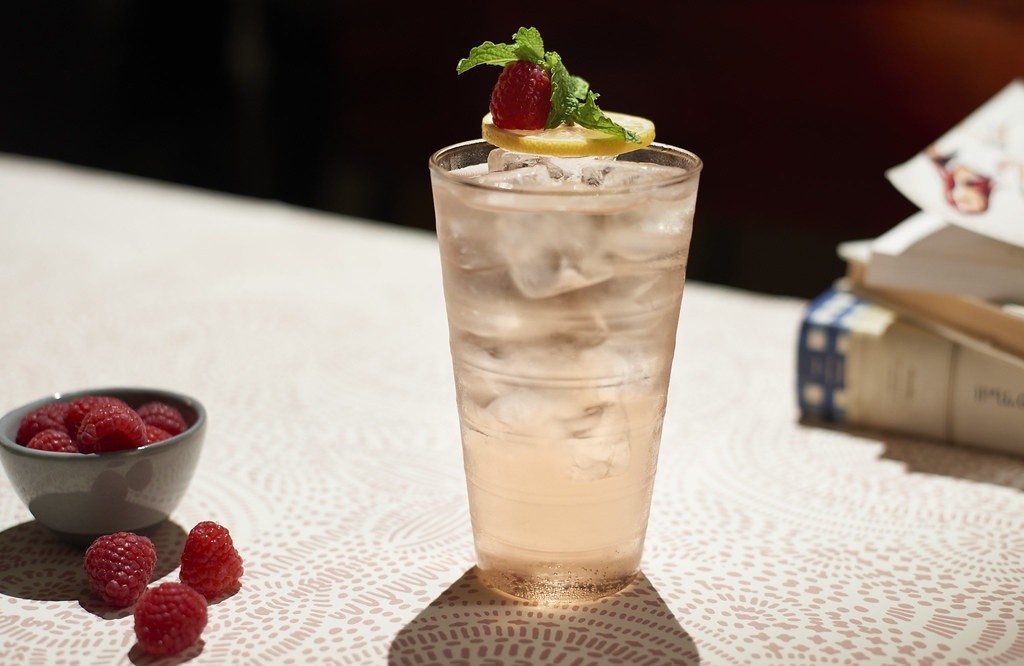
[429,137,704,603]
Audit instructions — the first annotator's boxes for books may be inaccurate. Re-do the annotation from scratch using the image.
[794,77,1024,458]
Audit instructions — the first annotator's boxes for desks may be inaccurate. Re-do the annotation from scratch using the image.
[0,154,1024,665]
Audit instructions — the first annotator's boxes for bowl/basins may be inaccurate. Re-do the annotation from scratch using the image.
[0,388,207,534]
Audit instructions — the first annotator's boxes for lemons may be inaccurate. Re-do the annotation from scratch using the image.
[481,109,655,157]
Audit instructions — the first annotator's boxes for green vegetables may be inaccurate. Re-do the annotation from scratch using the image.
[457,26,644,146]
